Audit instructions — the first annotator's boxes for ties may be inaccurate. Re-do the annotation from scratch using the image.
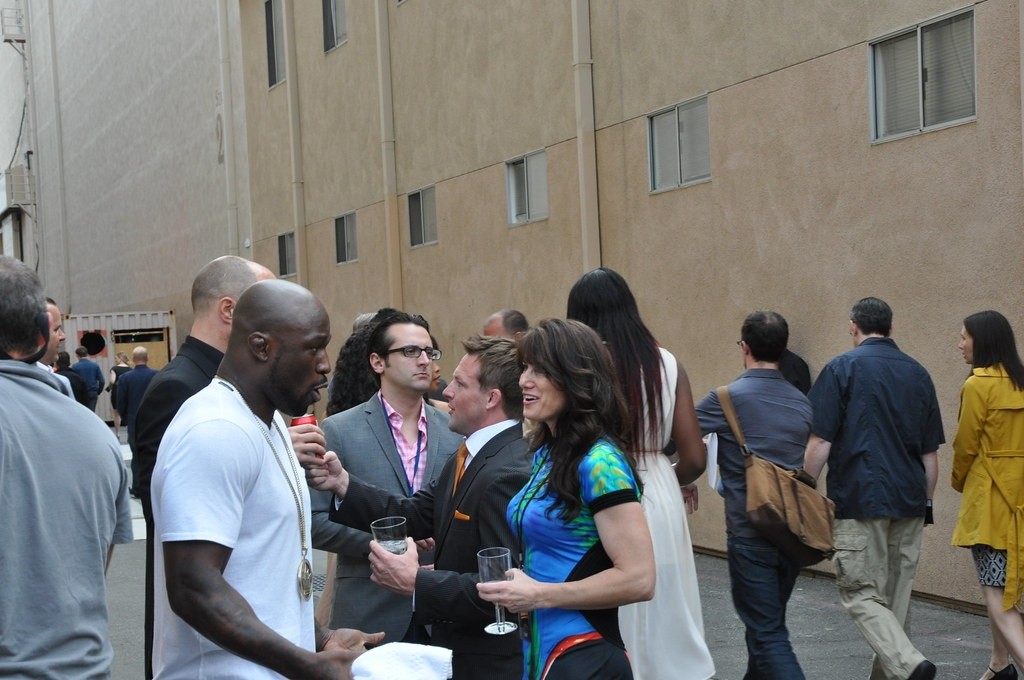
[452,442,469,499]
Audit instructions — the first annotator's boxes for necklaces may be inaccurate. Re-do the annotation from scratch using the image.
[516,447,554,572]
[216,374,314,600]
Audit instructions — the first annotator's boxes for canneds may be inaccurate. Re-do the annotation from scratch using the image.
[290,413,323,459]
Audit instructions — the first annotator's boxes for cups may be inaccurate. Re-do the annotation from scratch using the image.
[370,516,408,555]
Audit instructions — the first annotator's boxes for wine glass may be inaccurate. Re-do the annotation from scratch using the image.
[477,547,519,634]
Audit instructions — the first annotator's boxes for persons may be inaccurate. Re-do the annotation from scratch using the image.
[951,310,1024,680]
[663,311,812,680]
[0,256,535,680]
[477,317,655,680]
[803,296,947,680]
[568,268,716,680]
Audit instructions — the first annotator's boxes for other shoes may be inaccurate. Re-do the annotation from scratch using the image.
[907,659,935,680]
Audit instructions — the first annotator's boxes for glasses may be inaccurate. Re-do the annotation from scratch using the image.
[431,348,442,360]
[737,339,746,346]
[380,346,434,359]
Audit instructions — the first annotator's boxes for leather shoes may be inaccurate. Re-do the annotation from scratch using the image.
[987,663,1018,680]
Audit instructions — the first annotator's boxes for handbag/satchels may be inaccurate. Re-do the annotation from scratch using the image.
[743,453,836,568]
